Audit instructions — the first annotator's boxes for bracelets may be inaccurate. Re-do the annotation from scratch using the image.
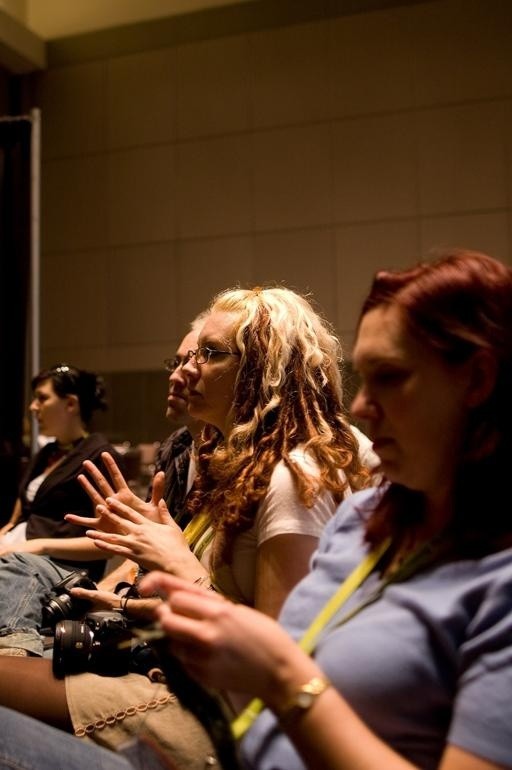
[274,671,333,739]
[192,569,218,592]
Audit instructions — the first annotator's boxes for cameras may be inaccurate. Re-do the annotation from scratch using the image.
[53,610,131,677]
[42,572,99,629]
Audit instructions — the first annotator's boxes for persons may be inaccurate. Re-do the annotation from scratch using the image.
[0,246,511,770]
[1,307,207,654]
[1,282,383,759]
[1,361,126,579]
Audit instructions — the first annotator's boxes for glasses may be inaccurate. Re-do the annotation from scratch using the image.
[52,364,77,388]
[163,353,189,372]
[186,344,242,365]
